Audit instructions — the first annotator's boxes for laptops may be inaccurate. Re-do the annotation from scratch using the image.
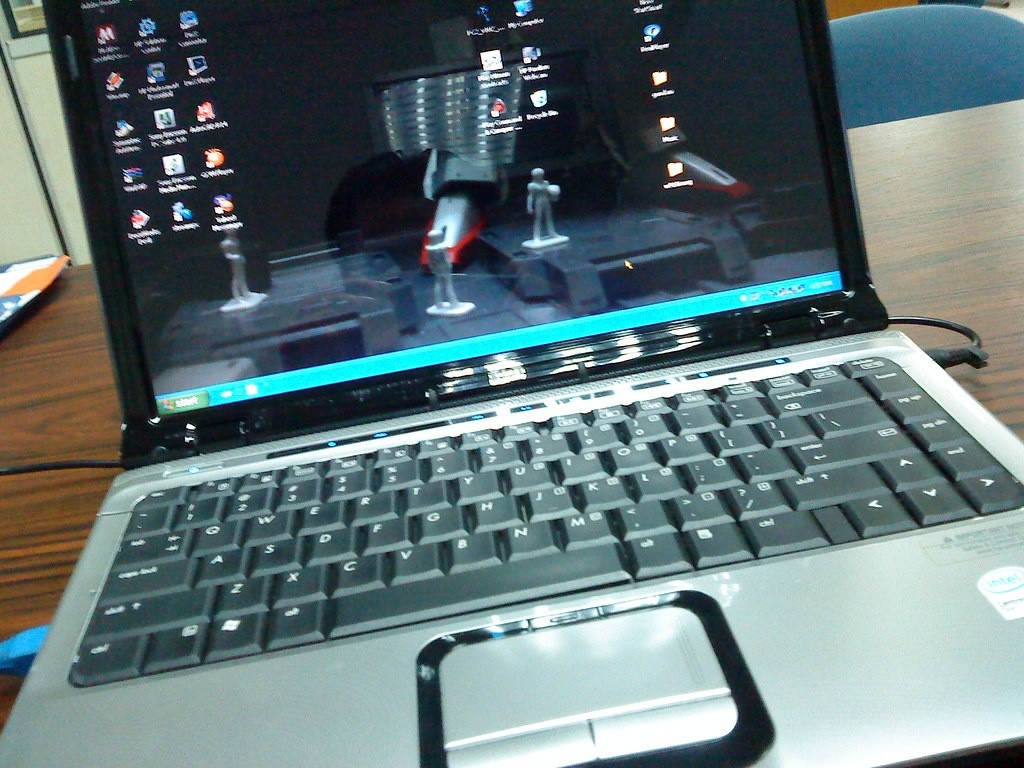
[0,0,1024,768]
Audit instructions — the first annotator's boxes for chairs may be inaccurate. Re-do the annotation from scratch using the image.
[827,6,1023,127]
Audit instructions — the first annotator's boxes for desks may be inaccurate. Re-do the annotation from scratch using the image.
[0,98,1024,767]
